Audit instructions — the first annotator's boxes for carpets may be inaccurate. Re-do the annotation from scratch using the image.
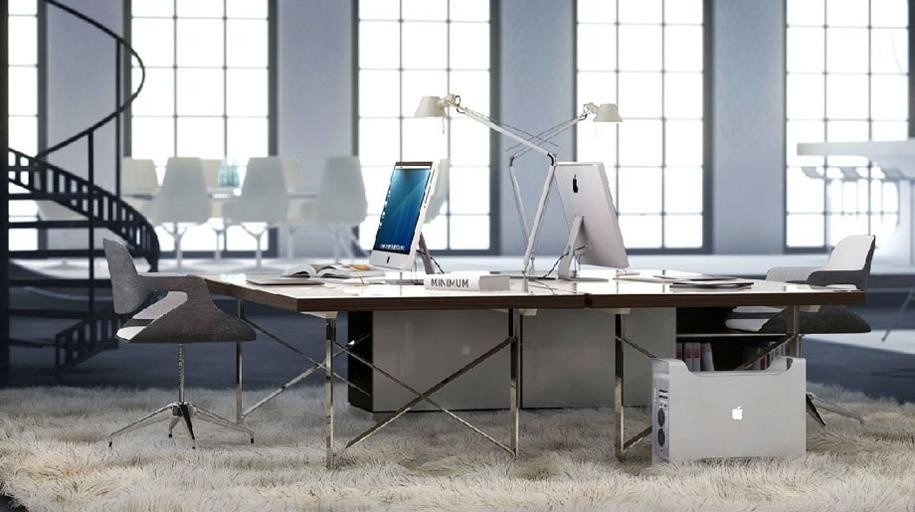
[1,382,915,511]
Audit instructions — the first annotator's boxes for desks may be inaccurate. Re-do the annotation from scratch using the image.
[192,270,864,468]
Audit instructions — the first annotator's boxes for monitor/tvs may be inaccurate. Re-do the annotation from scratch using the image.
[553,161,628,281]
[369,160,449,285]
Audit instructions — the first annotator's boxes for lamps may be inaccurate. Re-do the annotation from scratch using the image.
[490,104,643,281]
[414,95,555,293]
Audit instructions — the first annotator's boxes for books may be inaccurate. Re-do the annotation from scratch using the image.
[423,271,510,293]
[676,341,788,373]
[278,262,387,279]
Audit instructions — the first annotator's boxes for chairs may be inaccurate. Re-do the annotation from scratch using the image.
[102,239,256,447]
[118,155,369,271]
[716,233,878,425]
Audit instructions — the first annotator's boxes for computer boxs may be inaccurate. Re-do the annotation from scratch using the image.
[650,356,806,465]
